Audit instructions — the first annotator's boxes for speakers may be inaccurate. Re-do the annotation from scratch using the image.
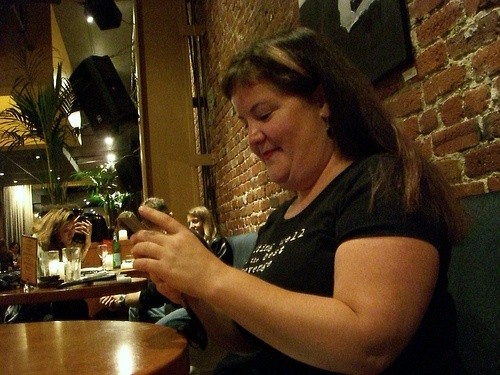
[69,56,135,131]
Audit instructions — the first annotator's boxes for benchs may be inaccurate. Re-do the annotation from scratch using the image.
[224,191,499,375]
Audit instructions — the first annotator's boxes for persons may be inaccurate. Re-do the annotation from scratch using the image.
[0,196,233,332]
[128,26,468,375]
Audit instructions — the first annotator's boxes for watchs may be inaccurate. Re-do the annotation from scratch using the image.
[118,294,126,310]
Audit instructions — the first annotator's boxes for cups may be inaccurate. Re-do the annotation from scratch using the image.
[45,251,60,282]
[61,247,82,281]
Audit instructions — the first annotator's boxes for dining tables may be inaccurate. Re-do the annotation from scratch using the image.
[0,275,148,304]
[0,320,192,375]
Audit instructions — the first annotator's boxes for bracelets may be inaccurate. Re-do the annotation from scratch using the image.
[82,246,89,252]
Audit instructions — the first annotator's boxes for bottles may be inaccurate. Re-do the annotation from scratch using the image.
[111,232,122,268]
[105,253,113,271]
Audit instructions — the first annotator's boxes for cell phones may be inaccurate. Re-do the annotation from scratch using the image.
[119,210,145,232]
[78,216,89,231]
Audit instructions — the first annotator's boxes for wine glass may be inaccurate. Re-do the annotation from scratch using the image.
[97,245,108,271]
